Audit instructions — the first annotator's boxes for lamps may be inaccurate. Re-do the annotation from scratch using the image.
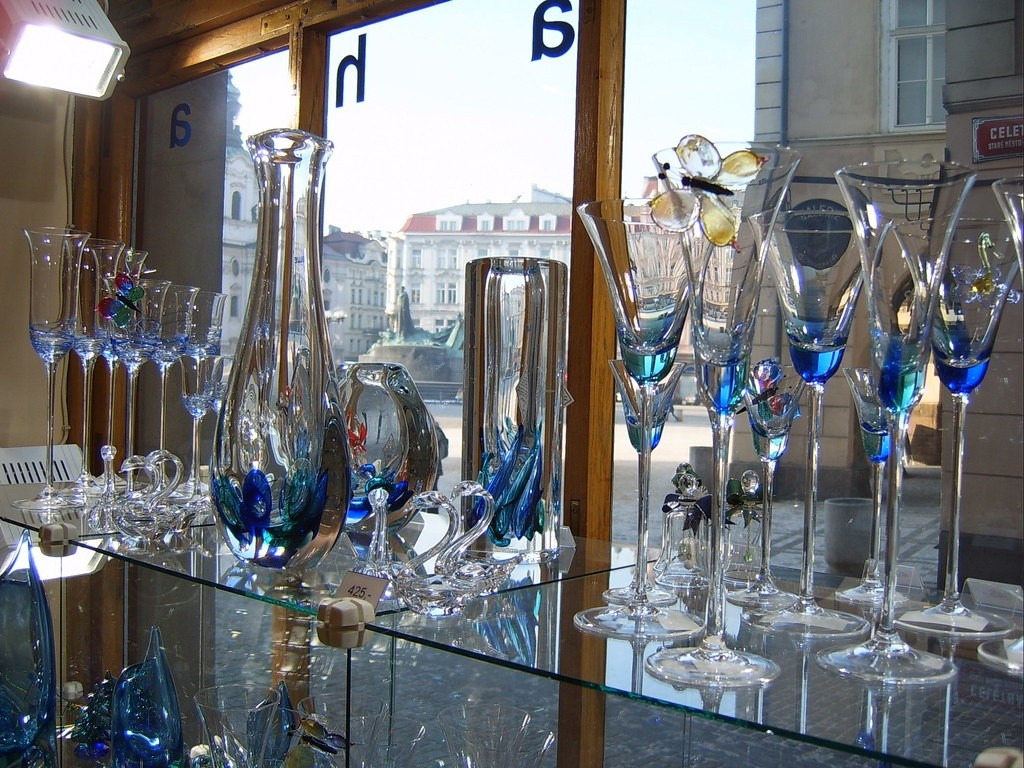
[0,0,131,101]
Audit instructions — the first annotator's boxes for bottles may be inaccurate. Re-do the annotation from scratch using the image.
[110,626,183,768]
[0,528,57,768]
[213,128,351,568]
[338,360,440,536]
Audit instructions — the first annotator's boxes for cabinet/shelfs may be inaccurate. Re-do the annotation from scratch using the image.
[0,480,1023,768]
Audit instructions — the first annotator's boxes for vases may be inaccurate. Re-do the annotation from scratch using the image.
[728,461,764,485]
[690,447,713,494]
[826,496,875,579]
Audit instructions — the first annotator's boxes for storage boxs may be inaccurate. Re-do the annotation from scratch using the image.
[907,402,942,468]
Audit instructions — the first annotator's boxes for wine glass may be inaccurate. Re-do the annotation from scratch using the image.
[601,359,687,608]
[574,197,705,643]
[815,160,976,684]
[13,226,210,513]
[747,209,871,640]
[978,175,1024,679]
[834,364,914,608]
[646,141,802,687]
[726,364,806,610]
[893,218,1020,637]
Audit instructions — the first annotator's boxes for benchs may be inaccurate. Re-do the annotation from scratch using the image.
[415,383,463,405]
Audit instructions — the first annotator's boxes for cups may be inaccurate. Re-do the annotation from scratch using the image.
[460,256,568,554]
[297,693,389,768]
[513,724,556,768]
[367,715,426,768]
[437,702,531,768]
[192,684,281,768]
[656,474,712,589]
[721,467,766,586]
[652,461,700,573]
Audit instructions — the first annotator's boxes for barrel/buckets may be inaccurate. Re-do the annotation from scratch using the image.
[823,497,874,576]
